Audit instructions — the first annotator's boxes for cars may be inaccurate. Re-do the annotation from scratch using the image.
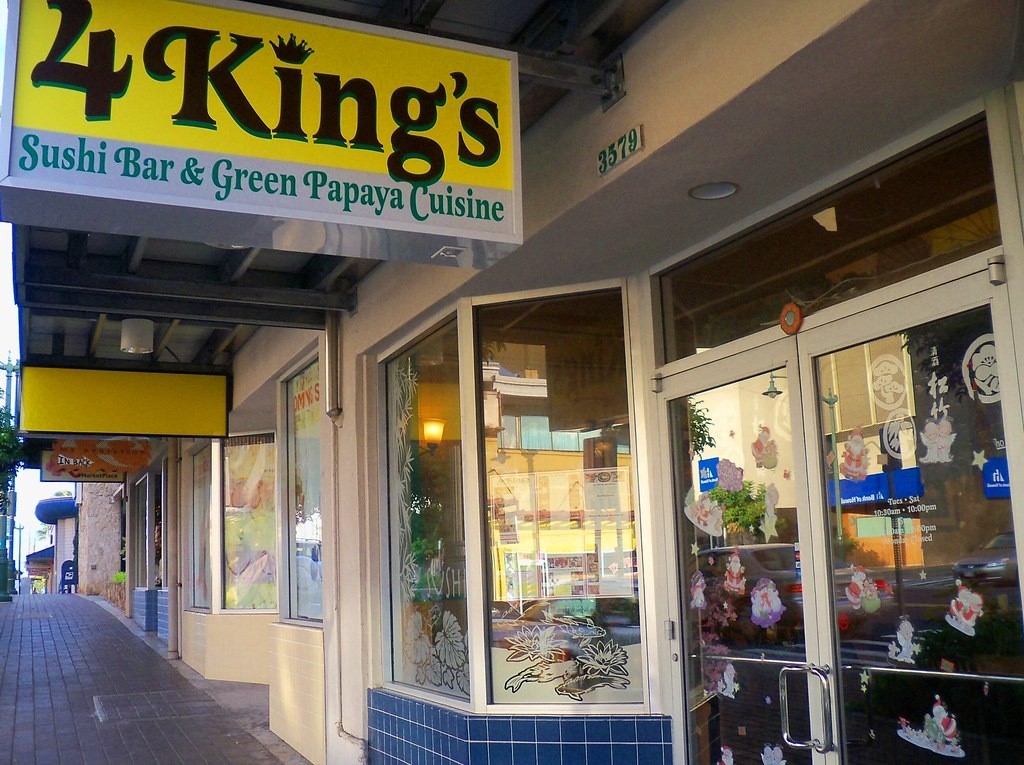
[952,532,1021,590]
[690,542,897,647]
[493,577,641,665]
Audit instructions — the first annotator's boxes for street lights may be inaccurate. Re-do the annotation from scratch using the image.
[762,372,845,563]
[491,439,542,597]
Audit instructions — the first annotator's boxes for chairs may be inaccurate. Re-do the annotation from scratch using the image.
[58,560,77,593]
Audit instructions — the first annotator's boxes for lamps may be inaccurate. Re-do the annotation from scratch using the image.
[120,317,153,354]
[419,417,447,457]
